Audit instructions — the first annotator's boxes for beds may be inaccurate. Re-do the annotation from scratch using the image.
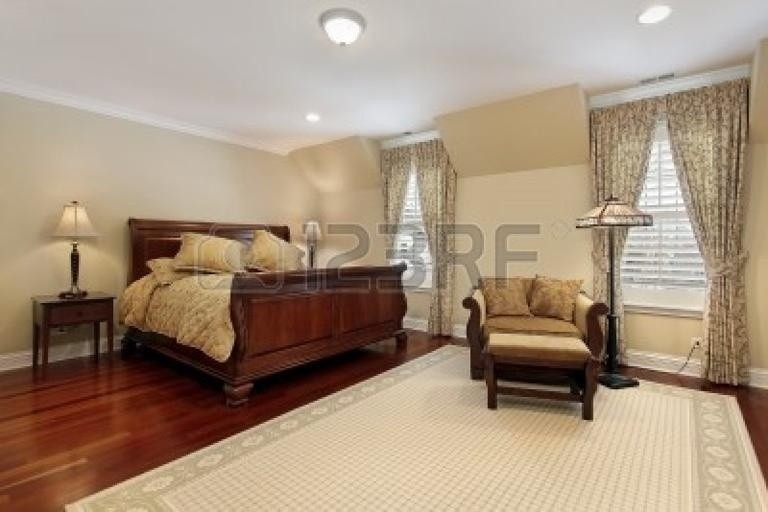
[116,212,412,411]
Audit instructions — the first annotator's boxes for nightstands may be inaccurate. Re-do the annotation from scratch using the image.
[29,289,117,385]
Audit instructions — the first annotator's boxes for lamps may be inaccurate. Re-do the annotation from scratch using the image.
[317,5,370,49]
[46,195,104,301]
[300,217,326,269]
[572,190,655,389]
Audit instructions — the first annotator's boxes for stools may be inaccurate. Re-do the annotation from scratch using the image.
[482,330,599,421]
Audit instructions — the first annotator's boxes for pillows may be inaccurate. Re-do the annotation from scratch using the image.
[527,273,583,324]
[170,230,245,278]
[144,253,205,286]
[241,229,307,276]
[475,275,532,321]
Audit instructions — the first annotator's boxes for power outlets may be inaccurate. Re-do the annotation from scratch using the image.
[688,335,705,350]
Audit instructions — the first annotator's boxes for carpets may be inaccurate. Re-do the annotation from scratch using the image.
[57,339,768,512]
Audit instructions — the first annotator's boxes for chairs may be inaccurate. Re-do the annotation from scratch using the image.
[459,271,608,392]
